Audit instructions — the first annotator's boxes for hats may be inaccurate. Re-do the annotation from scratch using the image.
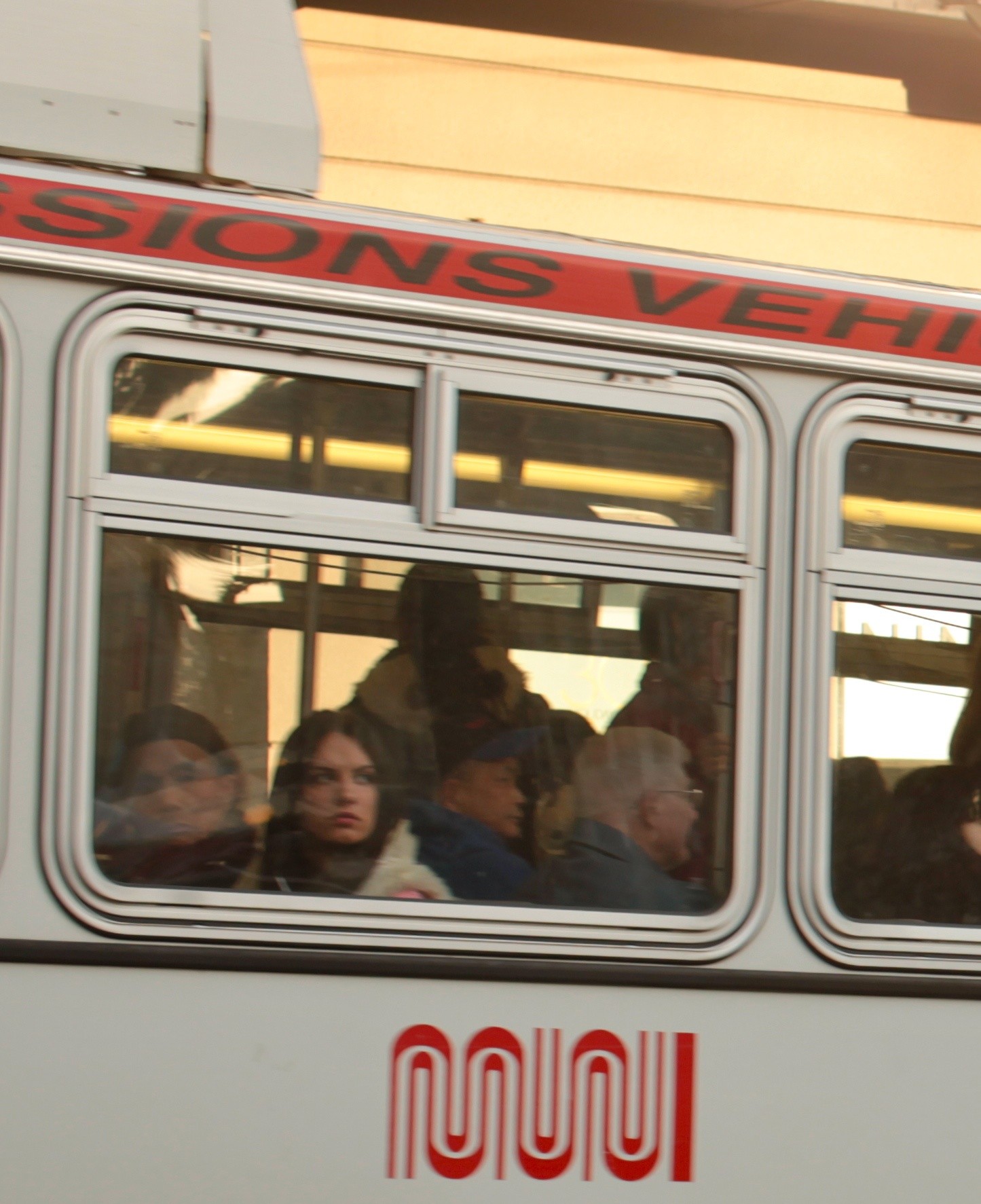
[123,705,239,778]
[431,708,538,778]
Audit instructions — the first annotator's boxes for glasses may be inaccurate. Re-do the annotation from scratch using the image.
[635,786,705,808]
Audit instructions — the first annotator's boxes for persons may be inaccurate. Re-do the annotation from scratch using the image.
[884,762,981,929]
[604,582,738,798]
[112,701,260,892]
[550,727,725,916]
[252,711,454,916]
[389,717,563,914]
[517,709,598,815]
[339,562,548,799]
